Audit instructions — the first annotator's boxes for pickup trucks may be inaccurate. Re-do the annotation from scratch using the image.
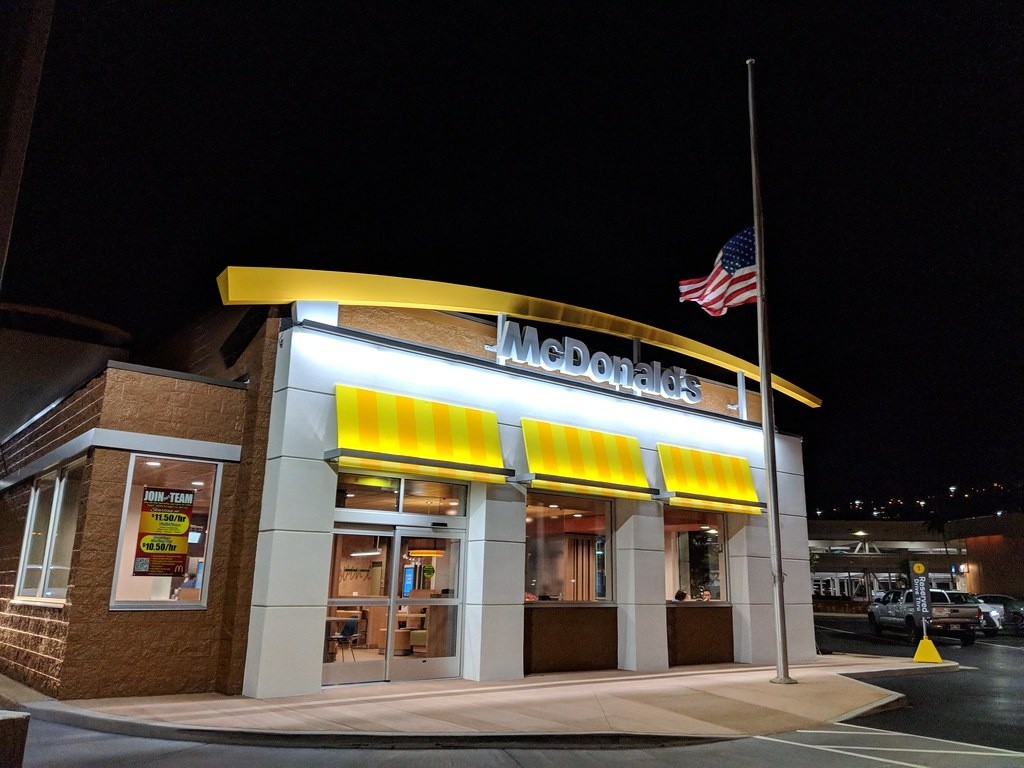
[866,587,983,647]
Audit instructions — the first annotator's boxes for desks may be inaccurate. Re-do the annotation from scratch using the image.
[336,610,362,649]
[326,616,358,624]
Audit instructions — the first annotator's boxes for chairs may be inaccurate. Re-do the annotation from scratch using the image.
[328,615,358,663]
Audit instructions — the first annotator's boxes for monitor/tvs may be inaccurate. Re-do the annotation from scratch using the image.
[402,565,424,599]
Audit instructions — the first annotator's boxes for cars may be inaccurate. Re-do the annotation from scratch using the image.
[965,594,1022,626]
[945,589,1000,638]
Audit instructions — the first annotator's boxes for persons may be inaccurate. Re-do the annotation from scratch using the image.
[675,589,687,601]
[700,588,712,601]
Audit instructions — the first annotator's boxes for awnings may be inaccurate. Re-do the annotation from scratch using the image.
[332,382,506,484]
[520,417,652,501]
[656,442,762,515]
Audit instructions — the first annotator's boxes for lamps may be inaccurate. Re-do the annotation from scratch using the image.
[350,536,382,557]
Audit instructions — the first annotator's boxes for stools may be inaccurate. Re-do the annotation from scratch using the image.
[377,628,411,656]
[409,630,427,656]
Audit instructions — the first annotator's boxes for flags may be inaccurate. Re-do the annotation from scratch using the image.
[679,225,758,316]
